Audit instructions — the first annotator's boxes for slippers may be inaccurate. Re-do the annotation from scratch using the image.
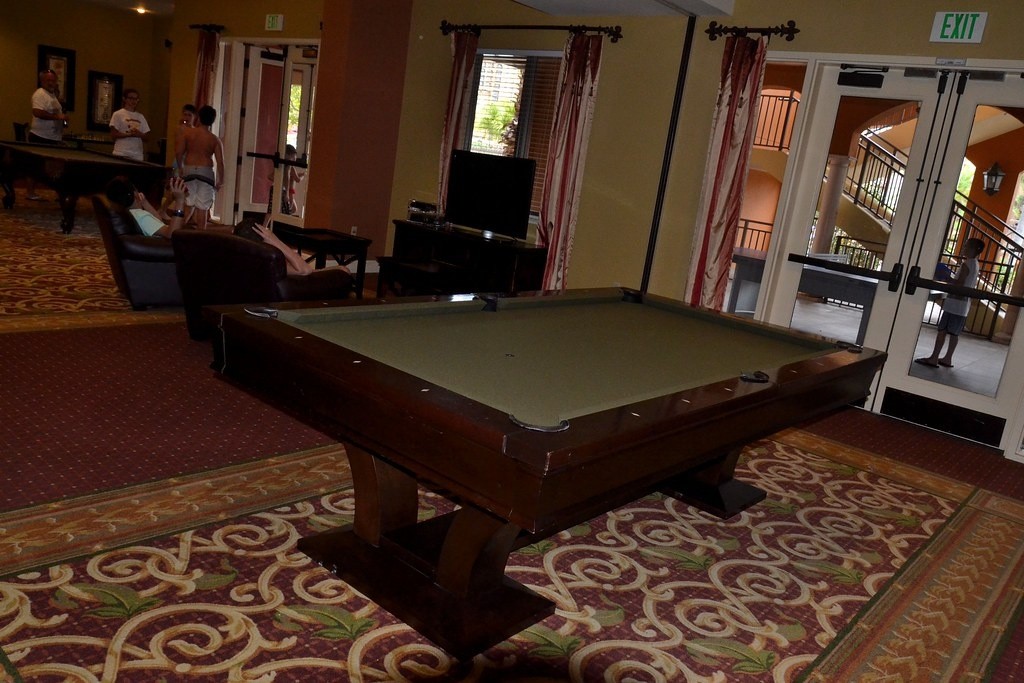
[938,359,954,367]
[914,358,939,368]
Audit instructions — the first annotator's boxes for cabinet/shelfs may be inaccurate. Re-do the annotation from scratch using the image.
[392,219,549,295]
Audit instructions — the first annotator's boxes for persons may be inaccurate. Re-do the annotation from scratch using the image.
[104,176,235,236]
[109,88,150,162]
[915,238,985,368]
[160,104,306,232]
[23,68,68,203]
[232,217,379,299]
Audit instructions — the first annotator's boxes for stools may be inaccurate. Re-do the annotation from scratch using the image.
[374,253,469,300]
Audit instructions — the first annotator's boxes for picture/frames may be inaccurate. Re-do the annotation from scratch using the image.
[37,43,74,111]
[86,70,124,132]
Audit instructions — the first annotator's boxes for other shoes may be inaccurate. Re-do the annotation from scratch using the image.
[25,194,39,200]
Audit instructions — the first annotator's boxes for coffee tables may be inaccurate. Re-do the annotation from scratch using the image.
[275,224,374,301]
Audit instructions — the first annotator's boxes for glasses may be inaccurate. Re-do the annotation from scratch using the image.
[126,96,138,101]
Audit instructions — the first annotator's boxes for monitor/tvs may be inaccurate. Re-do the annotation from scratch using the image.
[444,149,536,242]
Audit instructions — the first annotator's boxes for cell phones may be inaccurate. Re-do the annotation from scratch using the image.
[165,178,188,193]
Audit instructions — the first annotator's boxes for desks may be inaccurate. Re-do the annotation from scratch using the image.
[726,247,947,349]
[63,135,116,151]
[201,286,890,660]
[0,141,173,234]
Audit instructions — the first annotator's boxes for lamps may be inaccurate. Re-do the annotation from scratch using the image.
[982,161,1006,196]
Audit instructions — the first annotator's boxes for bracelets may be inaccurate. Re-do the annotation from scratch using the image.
[173,209,184,220]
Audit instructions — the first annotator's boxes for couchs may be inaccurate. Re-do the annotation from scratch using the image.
[173,229,354,340]
[89,188,187,315]
